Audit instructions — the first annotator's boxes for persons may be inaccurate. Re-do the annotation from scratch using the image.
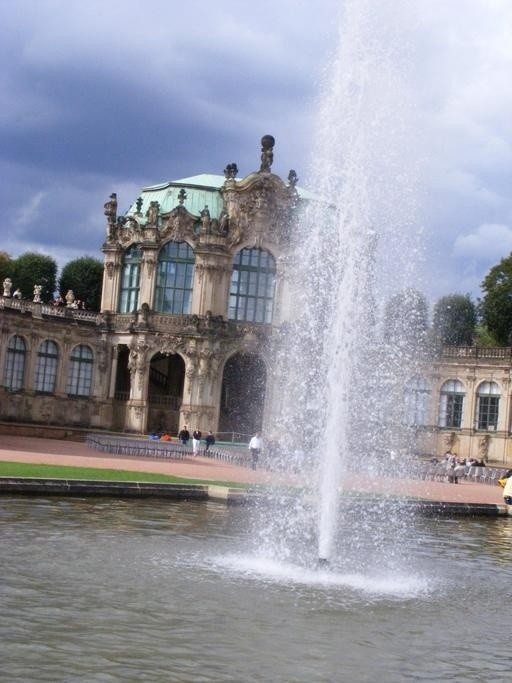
[12,287,86,310]
[199,204,212,234]
[360,441,512,512]
[149,425,287,475]
[102,192,118,230]
[146,200,159,226]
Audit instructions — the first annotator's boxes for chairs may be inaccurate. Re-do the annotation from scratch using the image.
[86,430,254,464]
[413,459,512,485]
